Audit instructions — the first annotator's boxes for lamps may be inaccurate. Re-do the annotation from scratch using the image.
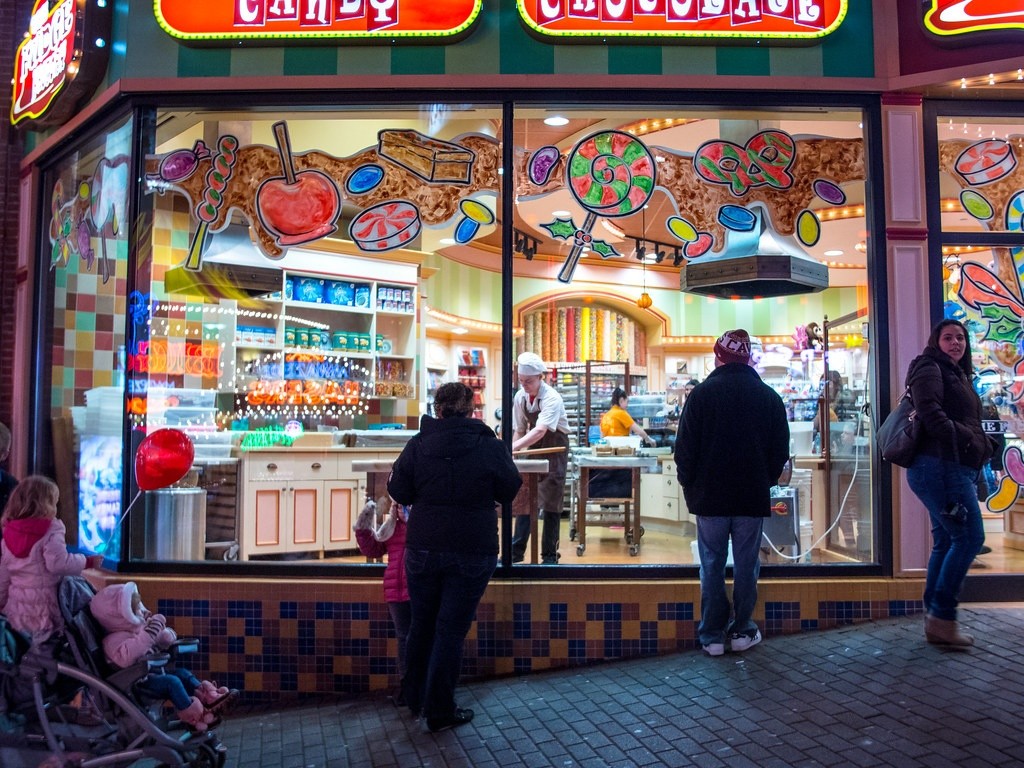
[637,207,651,307]
[544,112,569,125]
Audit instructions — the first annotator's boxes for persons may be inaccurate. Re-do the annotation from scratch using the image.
[0,419,19,514]
[811,371,869,556]
[497,350,571,565]
[597,386,658,525]
[0,475,105,713]
[90,581,240,732]
[353,467,413,707]
[386,382,523,734]
[683,379,702,399]
[673,328,790,656]
[804,323,825,350]
[874,318,1024,649]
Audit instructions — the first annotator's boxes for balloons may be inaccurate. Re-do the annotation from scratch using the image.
[134,428,194,491]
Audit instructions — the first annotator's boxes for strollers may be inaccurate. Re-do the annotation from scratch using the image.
[1,545,239,768]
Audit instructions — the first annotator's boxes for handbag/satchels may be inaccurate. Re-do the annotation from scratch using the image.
[876,359,942,469]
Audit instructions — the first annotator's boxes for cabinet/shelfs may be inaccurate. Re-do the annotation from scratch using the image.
[235,268,419,435]
[456,347,489,425]
[540,361,647,512]
[237,451,404,561]
[640,458,697,525]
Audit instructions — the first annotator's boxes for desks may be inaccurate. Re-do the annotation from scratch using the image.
[576,456,657,545]
[350,459,549,563]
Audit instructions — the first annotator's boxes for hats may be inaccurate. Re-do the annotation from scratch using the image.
[714,329,751,364]
[518,352,547,375]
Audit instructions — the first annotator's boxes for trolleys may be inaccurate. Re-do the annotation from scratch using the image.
[568,454,644,558]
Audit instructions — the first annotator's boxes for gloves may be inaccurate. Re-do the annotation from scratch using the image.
[144,614,166,639]
[356,501,376,530]
[159,626,176,645]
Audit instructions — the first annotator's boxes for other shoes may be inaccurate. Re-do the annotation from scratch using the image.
[497,552,523,564]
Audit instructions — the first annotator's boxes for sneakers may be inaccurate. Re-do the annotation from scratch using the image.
[703,643,724,655]
[731,629,761,651]
[427,709,474,732]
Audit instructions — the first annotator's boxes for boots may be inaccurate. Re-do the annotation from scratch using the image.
[195,681,232,711]
[178,697,222,734]
[924,614,974,645]
[397,678,408,705]
